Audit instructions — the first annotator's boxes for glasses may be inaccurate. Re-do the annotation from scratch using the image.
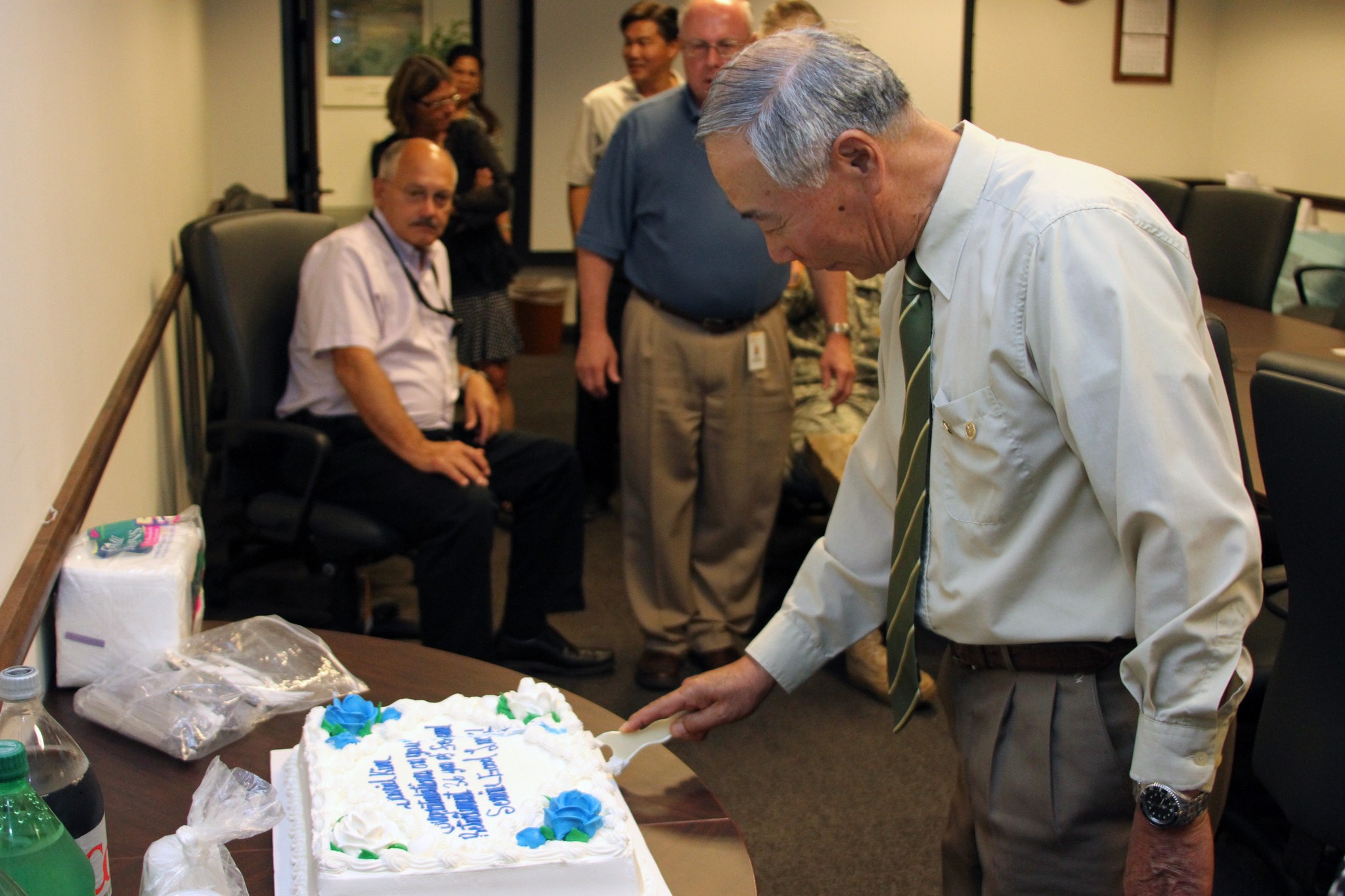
[684,37,749,58]
[419,93,460,111]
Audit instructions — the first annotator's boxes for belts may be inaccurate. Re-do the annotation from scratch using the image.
[632,285,782,334]
[950,638,1137,675]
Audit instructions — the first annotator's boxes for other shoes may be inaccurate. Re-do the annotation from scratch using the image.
[584,498,610,521]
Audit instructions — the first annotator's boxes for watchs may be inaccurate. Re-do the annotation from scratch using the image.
[1132,780,1215,832]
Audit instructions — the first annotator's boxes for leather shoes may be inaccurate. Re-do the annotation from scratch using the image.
[635,650,678,689]
[494,626,616,676]
[687,647,741,672]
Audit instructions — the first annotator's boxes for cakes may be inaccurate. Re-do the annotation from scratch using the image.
[282,677,648,896]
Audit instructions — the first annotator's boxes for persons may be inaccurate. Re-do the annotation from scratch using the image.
[564,0,938,711]
[369,54,521,434]
[621,33,1270,896]
[441,46,514,242]
[276,138,616,677]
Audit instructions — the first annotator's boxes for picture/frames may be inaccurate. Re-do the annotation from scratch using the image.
[1112,0,1176,85]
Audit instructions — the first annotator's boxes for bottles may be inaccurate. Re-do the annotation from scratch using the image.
[0,740,95,896]
[0,665,114,896]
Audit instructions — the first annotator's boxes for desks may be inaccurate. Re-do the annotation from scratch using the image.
[42,622,759,896]
[1203,293,1345,514]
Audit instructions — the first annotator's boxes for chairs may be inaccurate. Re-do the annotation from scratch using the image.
[180,179,1345,896]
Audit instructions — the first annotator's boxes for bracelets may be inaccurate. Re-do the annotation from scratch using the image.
[459,369,488,388]
[826,322,856,340]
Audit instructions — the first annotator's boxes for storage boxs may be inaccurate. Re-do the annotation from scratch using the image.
[55,517,202,686]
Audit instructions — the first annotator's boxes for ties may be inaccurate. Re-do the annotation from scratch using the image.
[886,249,933,733]
